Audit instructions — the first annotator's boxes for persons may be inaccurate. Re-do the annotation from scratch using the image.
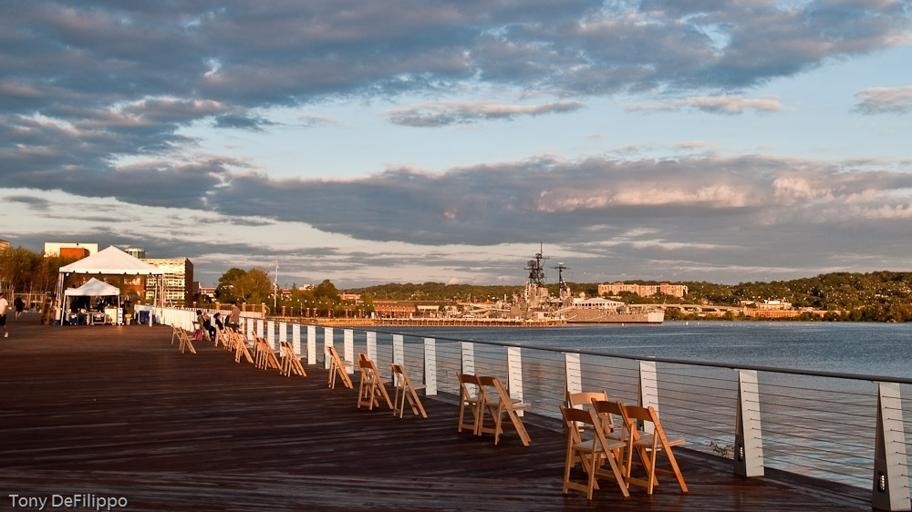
[189,299,241,343]
[70,296,118,325]
[13,294,25,321]
[0,291,10,338]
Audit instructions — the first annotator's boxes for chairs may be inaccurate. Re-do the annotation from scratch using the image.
[170,322,197,355]
[212,324,430,419]
[455,370,689,501]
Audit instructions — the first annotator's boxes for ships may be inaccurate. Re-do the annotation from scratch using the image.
[456,241,666,325]
